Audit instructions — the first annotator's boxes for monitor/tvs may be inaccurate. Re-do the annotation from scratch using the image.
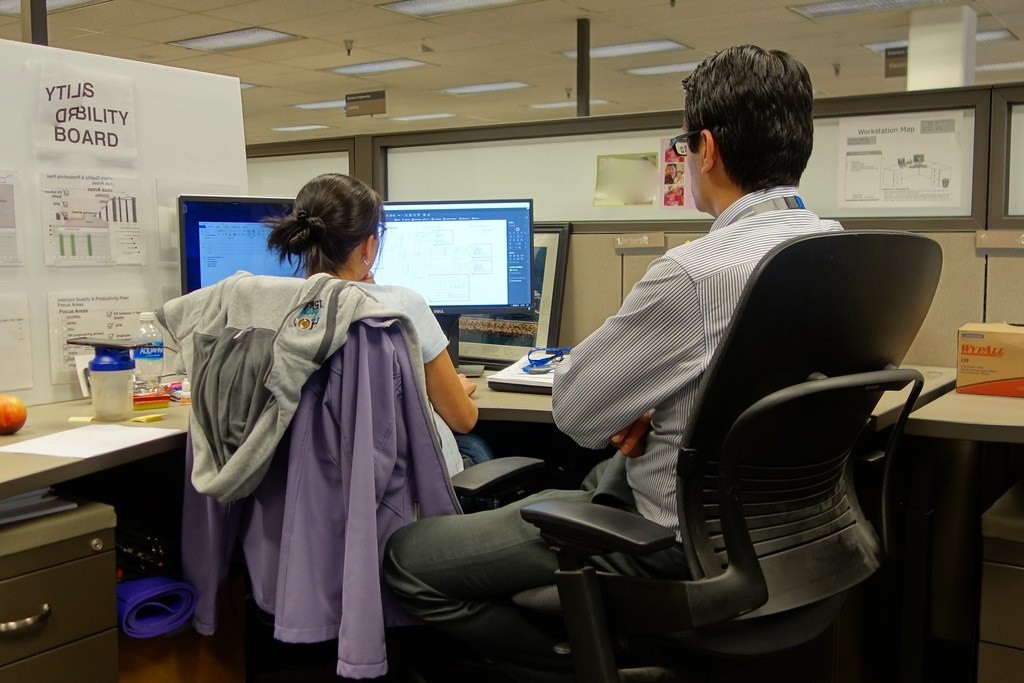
[176,194,309,297]
[369,199,534,375]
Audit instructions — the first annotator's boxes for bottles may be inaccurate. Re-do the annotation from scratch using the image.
[129,311,165,386]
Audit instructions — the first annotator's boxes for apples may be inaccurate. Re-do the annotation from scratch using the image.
[0,395,28,435]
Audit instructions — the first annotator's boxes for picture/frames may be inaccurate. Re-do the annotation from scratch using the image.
[458,221,573,363]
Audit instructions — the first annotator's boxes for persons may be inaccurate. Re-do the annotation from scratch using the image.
[664,163,684,185]
[268,173,494,514]
[378,44,845,683]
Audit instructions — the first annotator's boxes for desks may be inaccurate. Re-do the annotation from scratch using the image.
[0,366,1024,683]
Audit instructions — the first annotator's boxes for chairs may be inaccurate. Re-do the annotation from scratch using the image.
[150,271,544,683]
[523,230,943,683]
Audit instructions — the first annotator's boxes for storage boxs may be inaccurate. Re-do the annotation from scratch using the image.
[957,322,1024,398]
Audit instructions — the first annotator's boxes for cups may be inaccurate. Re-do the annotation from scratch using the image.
[88,347,136,423]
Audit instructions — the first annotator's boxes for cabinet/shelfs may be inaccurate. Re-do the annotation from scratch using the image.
[977,482,1024,683]
[0,502,122,683]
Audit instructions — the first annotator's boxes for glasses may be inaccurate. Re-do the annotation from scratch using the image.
[670,129,699,155]
[374,223,386,239]
